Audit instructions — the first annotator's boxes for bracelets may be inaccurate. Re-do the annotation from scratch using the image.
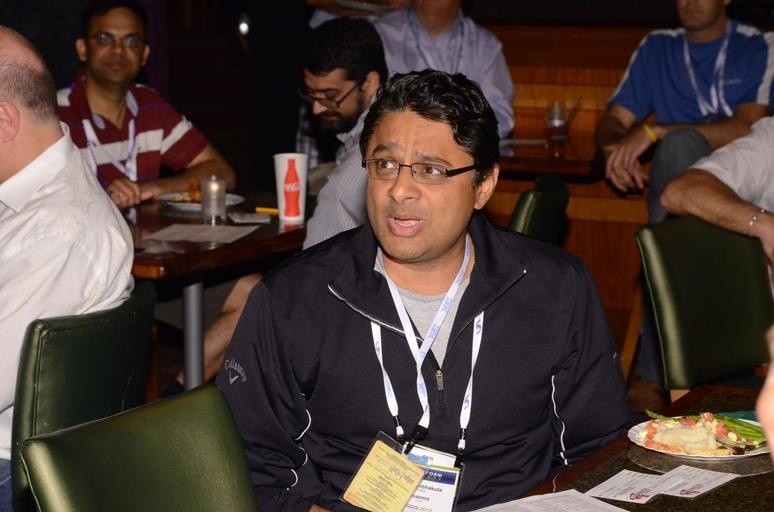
[746,208,766,236]
[642,123,658,145]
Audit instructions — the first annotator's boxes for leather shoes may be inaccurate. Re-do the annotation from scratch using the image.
[627,377,663,413]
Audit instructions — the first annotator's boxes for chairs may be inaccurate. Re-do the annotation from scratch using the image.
[508,189,569,235]
[637,212,774,402]
[16,383,260,512]
[11,277,158,511]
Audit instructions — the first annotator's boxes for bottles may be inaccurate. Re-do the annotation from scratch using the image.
[199,160,229,225]
[283,159,301,217]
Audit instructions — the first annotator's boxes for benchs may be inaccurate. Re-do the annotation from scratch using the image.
[480,64,702,383]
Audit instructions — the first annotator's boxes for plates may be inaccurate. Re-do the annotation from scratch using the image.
[338,0,390,12]
[626,415,771,461]
[159,192,245,213]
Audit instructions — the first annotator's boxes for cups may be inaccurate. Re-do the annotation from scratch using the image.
[272,153,309,223]
[544,99,570,161]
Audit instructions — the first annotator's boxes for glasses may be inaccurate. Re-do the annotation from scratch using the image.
[297,80,363,110]
[365,158,478,185]
[86,32,146,48]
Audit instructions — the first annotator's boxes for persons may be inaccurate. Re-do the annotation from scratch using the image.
[49,1,269,395]
[659,116,774,307]
[297,17,390,251]
[0,27,136,502]
[215,67,630,512]
[373,0,515,161]
[596,0,774,224]
[754,326,774,468]
